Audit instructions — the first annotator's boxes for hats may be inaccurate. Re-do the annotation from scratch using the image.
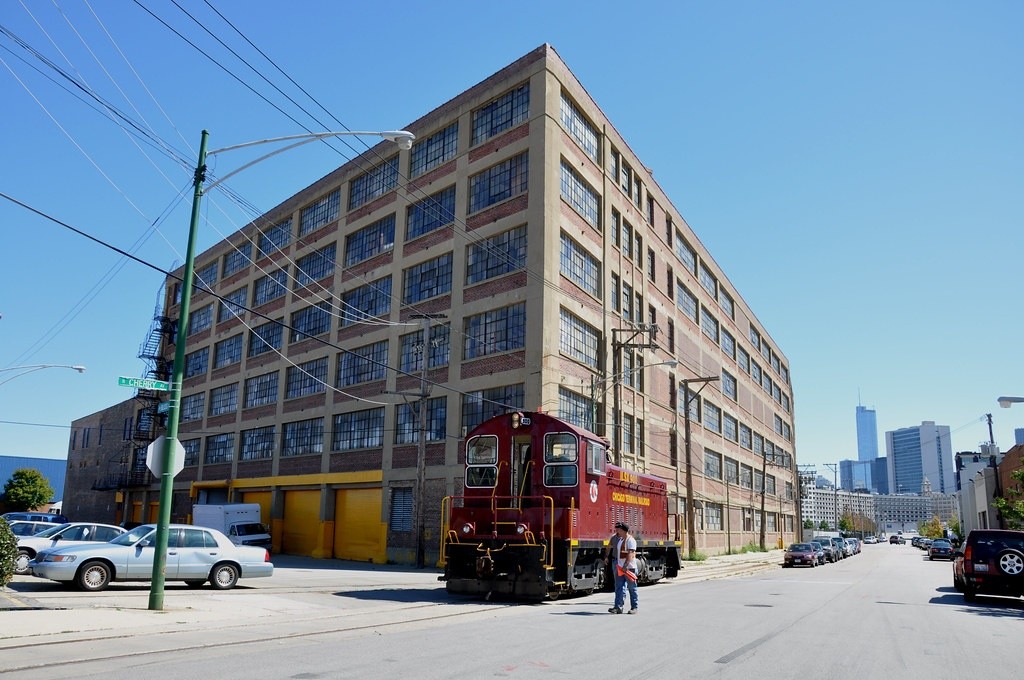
[615,522,629,532]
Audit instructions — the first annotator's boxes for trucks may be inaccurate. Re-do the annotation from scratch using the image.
[192,502,273,556]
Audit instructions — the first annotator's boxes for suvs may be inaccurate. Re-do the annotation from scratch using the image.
[952,528,1024,603]
[1,512,69,540]
[890,534,906,545]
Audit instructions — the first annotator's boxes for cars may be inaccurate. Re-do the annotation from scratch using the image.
[928,541,956,562]
[783,543,818,568]
[797,541,827,565]
[27,521,275,593]
[863,534,888,545]
[12,522,151,576]
[811,536,862,563]
[911,536,955,551]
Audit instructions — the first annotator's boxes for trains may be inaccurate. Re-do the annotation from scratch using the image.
[437,408,684,602]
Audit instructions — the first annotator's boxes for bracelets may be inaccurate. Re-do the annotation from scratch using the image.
[606,556,608,557]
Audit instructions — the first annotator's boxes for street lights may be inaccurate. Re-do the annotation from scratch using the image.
[148,128,419,612]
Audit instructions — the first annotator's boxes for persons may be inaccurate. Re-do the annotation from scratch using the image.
[608,522,639,614]
[605,522,626,605]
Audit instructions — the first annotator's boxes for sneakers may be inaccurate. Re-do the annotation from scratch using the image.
[628,608,637,614]
[608,606,623,614]
